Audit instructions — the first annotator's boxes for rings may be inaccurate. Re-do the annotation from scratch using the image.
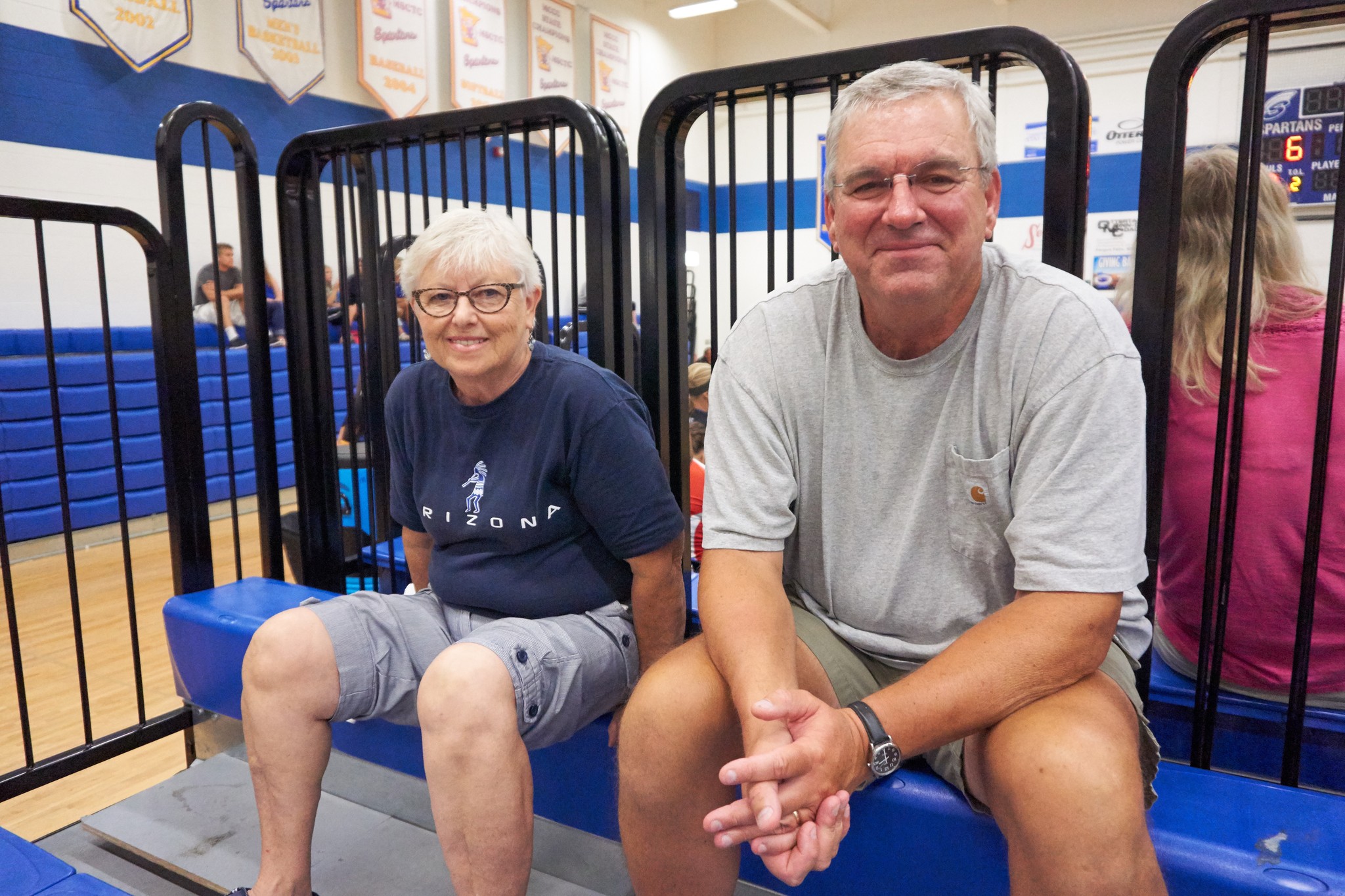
[793,811,802,827]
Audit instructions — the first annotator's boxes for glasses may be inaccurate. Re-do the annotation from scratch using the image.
[411,283,525,318]
[833,158,986,209]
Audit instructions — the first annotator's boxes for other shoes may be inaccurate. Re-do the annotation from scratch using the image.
[229,336,247,349]
[398,333,410,342]
[269,335,283,348]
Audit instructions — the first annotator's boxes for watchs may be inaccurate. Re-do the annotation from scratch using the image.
[848,701,902,780]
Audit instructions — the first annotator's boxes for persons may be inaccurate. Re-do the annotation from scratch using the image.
[193,244,287,348]
[338,374,360,441]
[224,204,713,896]
[617,56,1174,895]
[1110,145,1344,711]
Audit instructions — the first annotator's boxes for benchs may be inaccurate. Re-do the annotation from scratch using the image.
[0,314,588,356]
[0,542,1345,896]
